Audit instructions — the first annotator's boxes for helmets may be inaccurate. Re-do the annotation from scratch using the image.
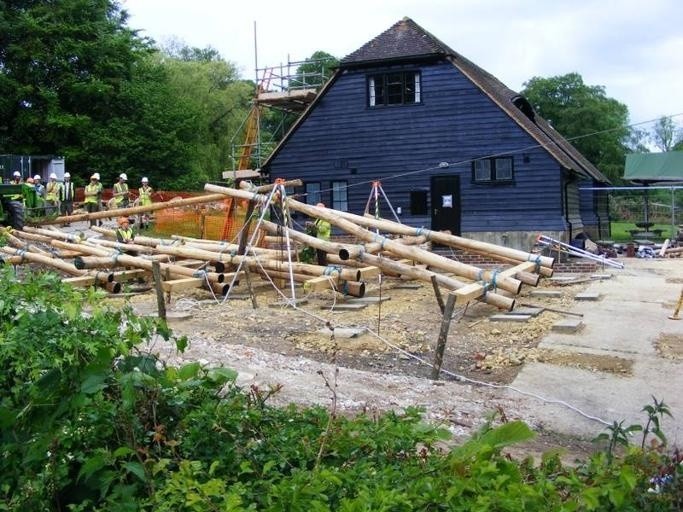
[141,176,149,183]
[119,217,130,225]
[90,173,100,181]
[14,170,71,180]
[316,203,325,207]
[119,172,128,181]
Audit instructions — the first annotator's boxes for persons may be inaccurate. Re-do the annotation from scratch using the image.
[82,176,100,226]
[59,172,75,228]
[92,172,104,211]
[32,175,44,198]
[112,172,130,209]
[8,170,23,202]
[44,173,60,215]
[305,203,330,266]
[138,176,153,230]
[116,217,138,256]
